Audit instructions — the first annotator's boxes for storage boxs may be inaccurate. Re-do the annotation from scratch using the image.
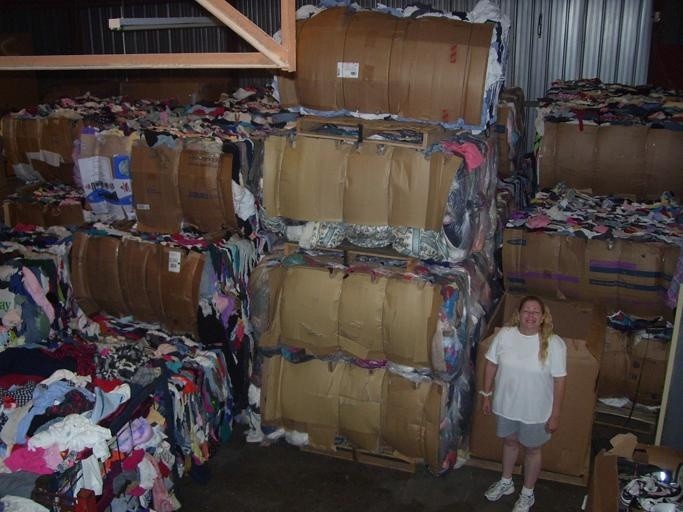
[502,293,674,406]
[261,351,458,476]
[72,232,206,344]
[119,79,229,111]
[259,253,455,375]
[503,226,681,323]
[590,446,682,510]
[469,330,599,477]
[492,88,525,178]
[280,8,492,126]
[541,122,683,200]
[264,134,456,244]
[2,200,83,232]
[0,113,104,186]
[85,151,243,234]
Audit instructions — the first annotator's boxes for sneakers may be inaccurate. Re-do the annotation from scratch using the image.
[484,480,515,501]
[512,493,535,512]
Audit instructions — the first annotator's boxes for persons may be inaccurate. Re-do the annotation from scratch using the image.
[480,296,567,508]
